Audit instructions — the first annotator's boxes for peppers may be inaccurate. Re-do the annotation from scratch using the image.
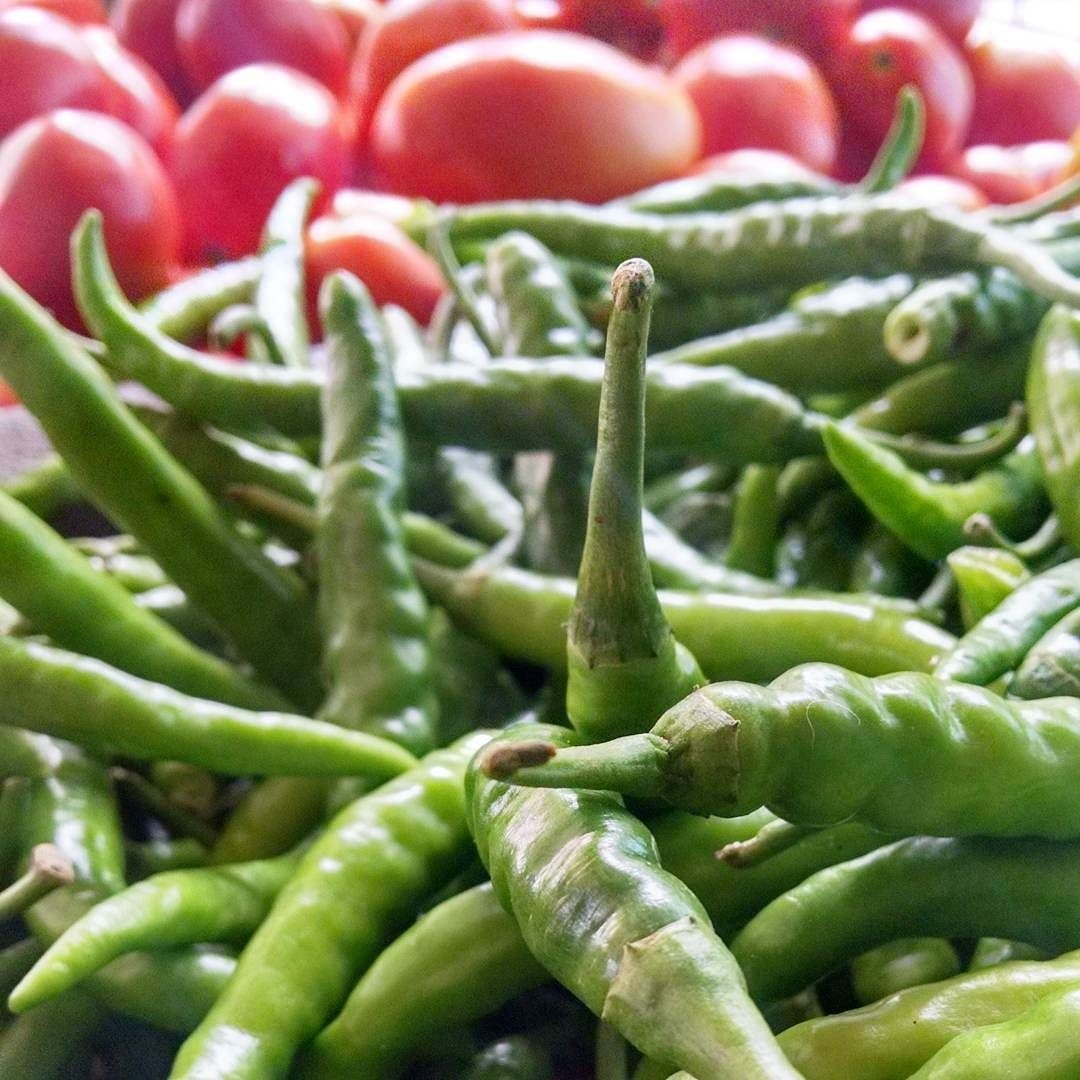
[0,86,1080,1079]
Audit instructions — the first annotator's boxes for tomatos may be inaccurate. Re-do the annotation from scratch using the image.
[0,0,1080,405]
